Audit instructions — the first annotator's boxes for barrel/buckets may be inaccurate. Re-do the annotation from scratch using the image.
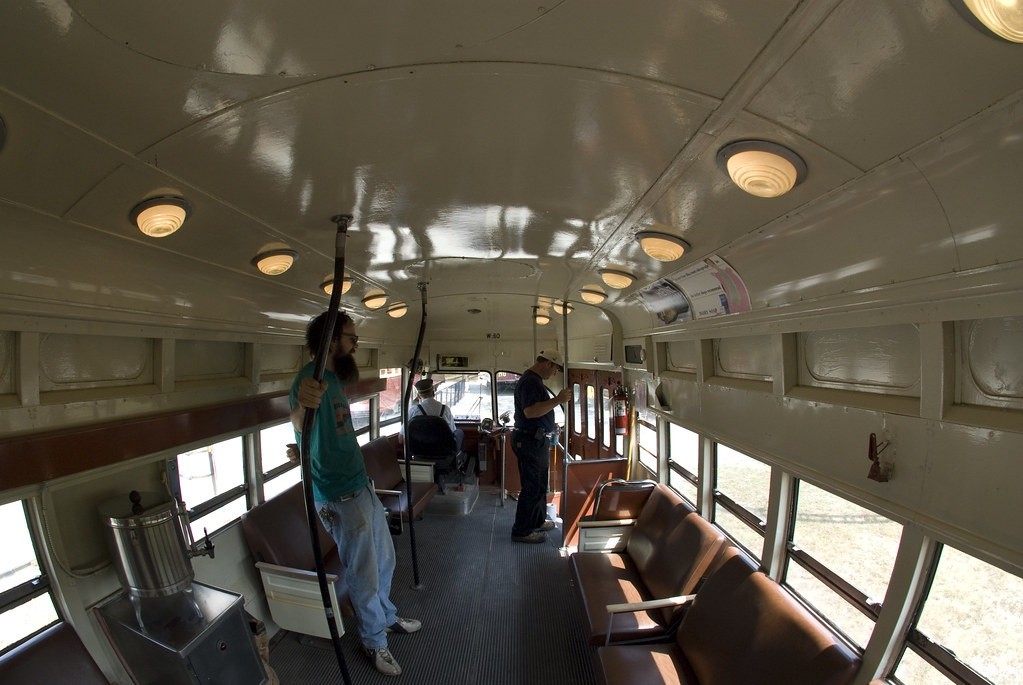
[97,491,194,602]
[546,503,557,528]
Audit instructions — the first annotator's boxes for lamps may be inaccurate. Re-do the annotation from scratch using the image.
[599,269,636,290]
[387,304,409,318]
[948,0,1023,45]
[251,251,299,275]
[360,295,390,309]
[578,289,607,304]
[130,197,192,239]
[319,278,355,296]
[551,303,574,315]
[530,315,552,326]
[636,232,691,263]
[717,140,807,198]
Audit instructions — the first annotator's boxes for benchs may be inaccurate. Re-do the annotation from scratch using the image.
[239,434,896,685]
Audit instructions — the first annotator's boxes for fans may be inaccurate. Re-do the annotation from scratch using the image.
[499,410,512,423]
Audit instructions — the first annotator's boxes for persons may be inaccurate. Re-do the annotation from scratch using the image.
[285,310,422,676]
[398,378,457,444]
[511,349,571,543]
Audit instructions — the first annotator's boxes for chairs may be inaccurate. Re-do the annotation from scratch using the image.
[408,415,465,473]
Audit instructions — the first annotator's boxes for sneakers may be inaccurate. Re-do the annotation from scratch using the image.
[386,616,422,634]
[366,648,402,676]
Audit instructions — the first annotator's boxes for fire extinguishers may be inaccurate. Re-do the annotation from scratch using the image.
[614,383,628,435]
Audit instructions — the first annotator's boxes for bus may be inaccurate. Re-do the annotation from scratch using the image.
[346,368,479,420]
[496,372,521,389]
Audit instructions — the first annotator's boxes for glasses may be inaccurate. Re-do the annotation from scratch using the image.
[341,332,359,342]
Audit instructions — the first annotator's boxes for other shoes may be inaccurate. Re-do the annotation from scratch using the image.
[511,519,555,544]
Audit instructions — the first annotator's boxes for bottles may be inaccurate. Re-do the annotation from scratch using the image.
[550,423,559,446]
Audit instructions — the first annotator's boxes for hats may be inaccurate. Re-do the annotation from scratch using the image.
[537,347,564,373]
[414,379,433,393]
